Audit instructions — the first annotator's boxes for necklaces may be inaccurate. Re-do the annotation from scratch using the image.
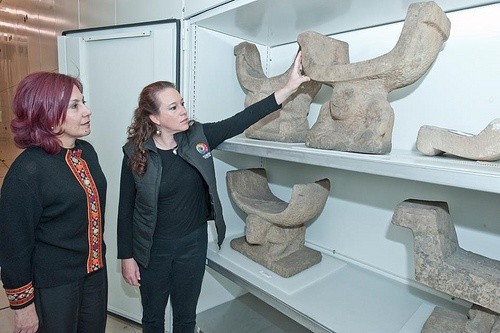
[152,134,182,156]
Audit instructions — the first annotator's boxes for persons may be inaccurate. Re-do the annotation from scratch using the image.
[115,49,311,333]
[0,71,109,333]
